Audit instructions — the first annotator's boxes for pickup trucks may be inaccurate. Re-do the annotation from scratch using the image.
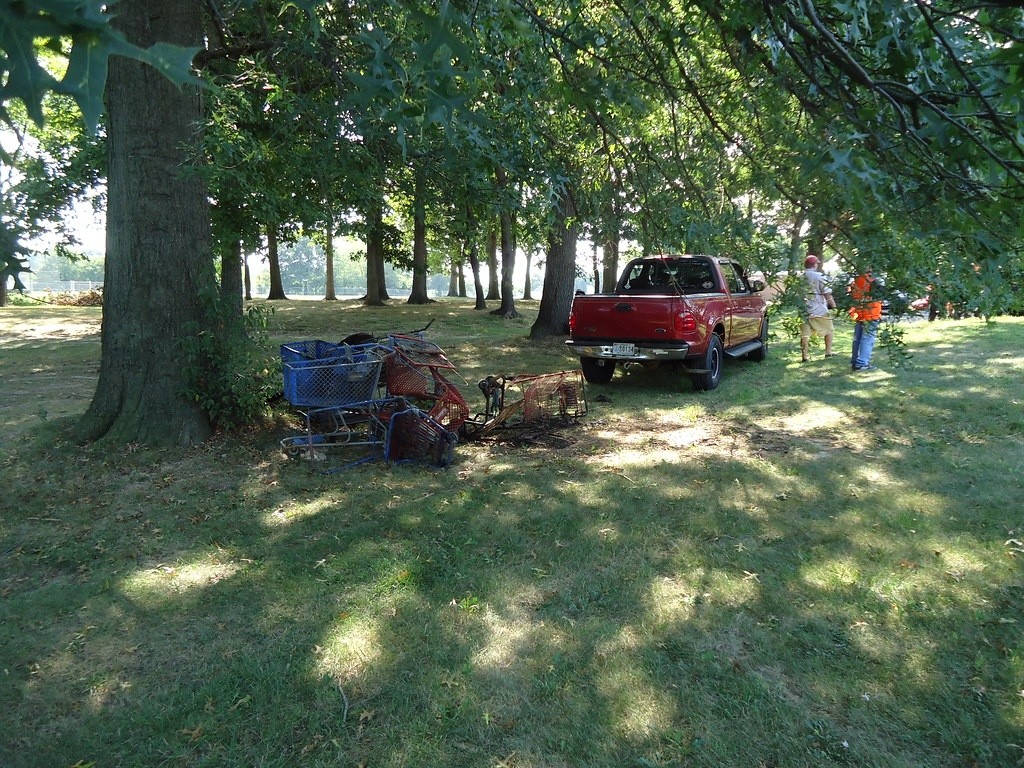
[564,254,771,392]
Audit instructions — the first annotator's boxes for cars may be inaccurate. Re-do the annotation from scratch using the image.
[827,273,909,314]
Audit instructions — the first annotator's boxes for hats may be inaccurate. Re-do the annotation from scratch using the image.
[806,255,821,266]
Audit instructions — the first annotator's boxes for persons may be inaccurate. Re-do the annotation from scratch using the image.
[797,255,837,363]
[847,263,882,372]
[927,285,946,321]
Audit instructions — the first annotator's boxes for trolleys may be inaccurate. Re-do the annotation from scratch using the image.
[270,317,588,476]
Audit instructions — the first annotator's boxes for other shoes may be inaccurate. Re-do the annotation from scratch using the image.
[854,365,876,372]
[825,354,836,358]
[803,359,809,362]
